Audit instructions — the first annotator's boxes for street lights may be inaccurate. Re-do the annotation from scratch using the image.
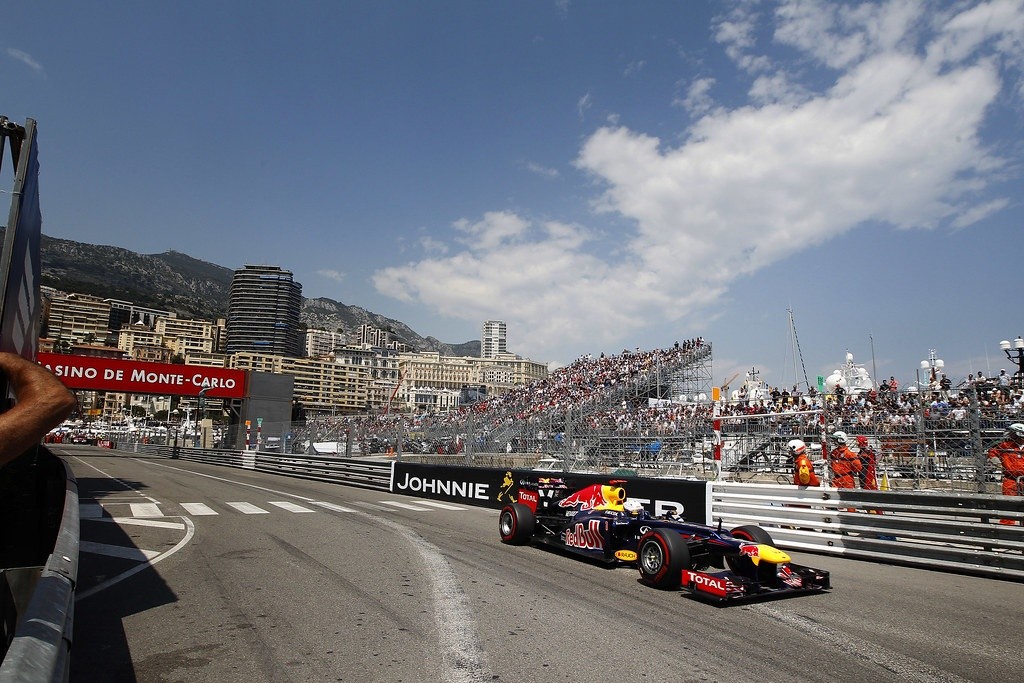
[173,410,180,449]
[117,407,126,437]
[868,331,876,393]
[193,387,213,447]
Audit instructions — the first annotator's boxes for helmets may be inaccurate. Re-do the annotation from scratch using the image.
[830,431,848,443]
[786,439,806,454]
[1005,423,1024,437]
[857,436,868,447]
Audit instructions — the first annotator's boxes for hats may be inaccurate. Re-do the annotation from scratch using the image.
[1001,368,1005,372]
[799,465,810,484]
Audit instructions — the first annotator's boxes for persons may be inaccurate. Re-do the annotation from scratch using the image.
[501,336,707,435]
[857,436,885,514]
[0,351,77,469]
[830,431,861,514]
[706,368,1024,435]
[290,395,502,455]
[622,500,656,519]
[781,438,819,531]
[988,423,1024,525]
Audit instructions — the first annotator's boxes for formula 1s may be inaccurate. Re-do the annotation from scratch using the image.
[499,479,833,608]
